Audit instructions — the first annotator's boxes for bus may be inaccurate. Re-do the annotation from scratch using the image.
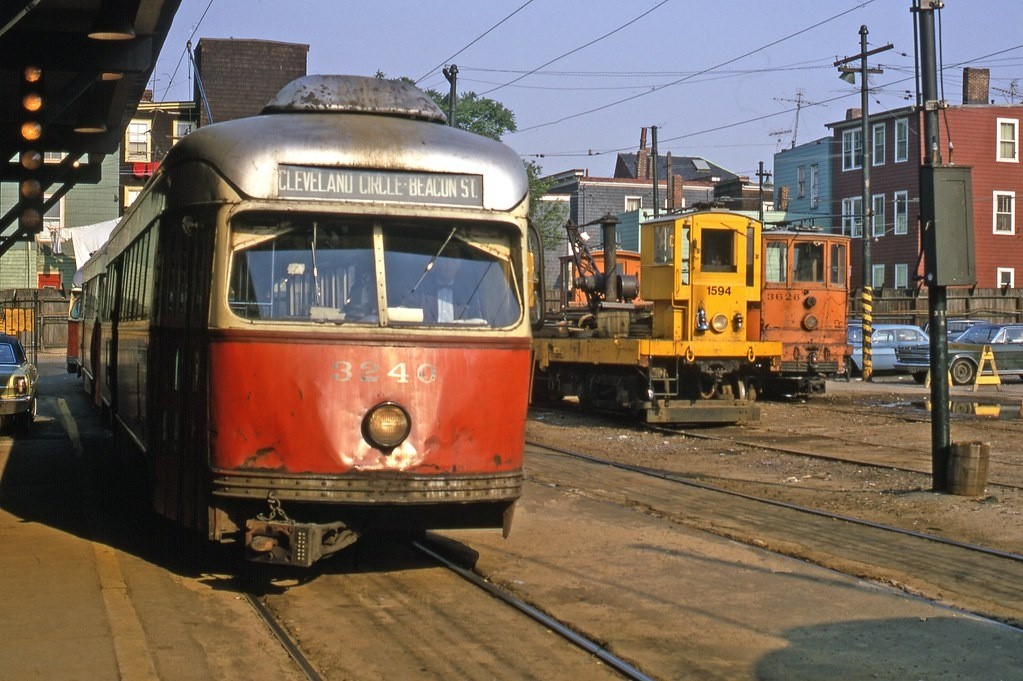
[67,74,546,568]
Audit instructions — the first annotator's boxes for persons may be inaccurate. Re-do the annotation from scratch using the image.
[398,241,479,323]
[706,243,725,265]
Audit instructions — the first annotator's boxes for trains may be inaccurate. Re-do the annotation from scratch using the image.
[532,193,782,422]
[559,225,851,399]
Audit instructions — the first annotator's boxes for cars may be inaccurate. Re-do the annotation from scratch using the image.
[924,318,991,341]
[0,334,39,423]
[893,324,1023,385]
[848,324,930,376]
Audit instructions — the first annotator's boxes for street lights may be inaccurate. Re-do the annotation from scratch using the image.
[837,68,872,380]
